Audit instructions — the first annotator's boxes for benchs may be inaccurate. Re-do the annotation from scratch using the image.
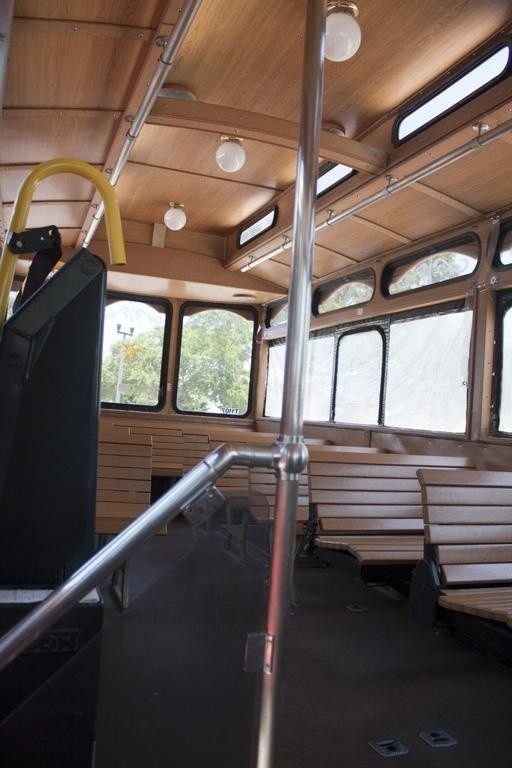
[308,452,474,600]
[102,379,255,472]
[248,444,393,581]
[416,468,511,629]
[96,434,167,612]
[209,432,337,561]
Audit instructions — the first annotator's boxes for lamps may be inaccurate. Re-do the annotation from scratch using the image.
[164,201,188,232]
[323,0,363,63]
[215,134,247,174]
